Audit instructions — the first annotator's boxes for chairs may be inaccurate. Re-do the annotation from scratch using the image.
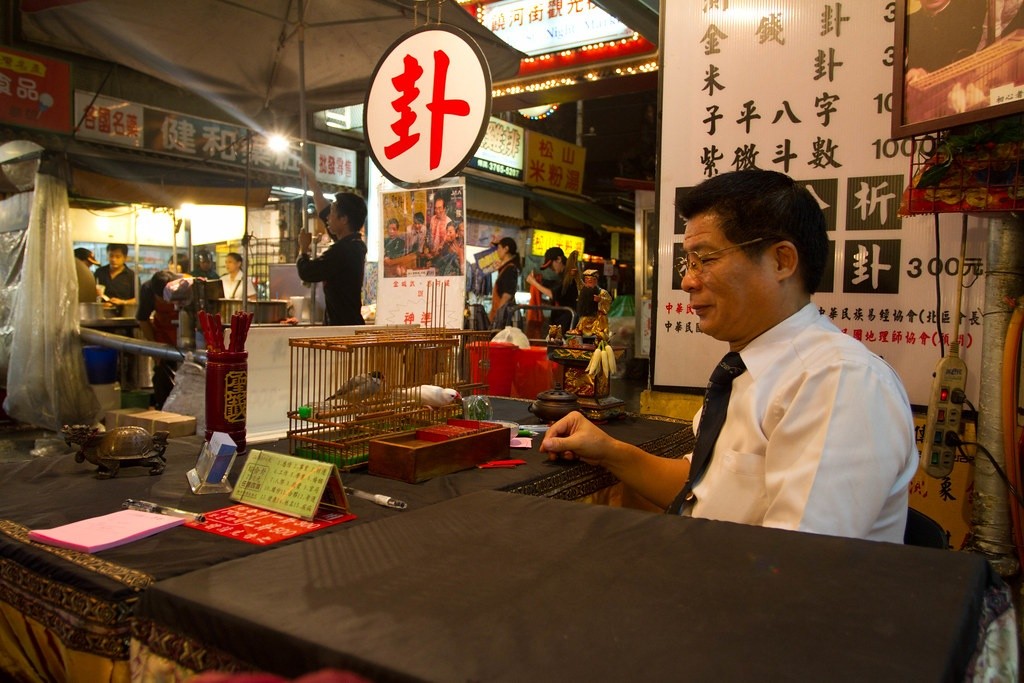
[904,507,948,550]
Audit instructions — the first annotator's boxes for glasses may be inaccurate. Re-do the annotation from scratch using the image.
[673,233,790,279]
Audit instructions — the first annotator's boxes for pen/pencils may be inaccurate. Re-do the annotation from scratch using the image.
[122,498,206,523]
[342,484,409,512]
[517,423,551,437]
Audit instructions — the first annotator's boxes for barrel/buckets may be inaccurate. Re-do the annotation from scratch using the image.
[90,383,121,411]
[84,345,118,384]
[514,346,557,399]
[466,341,517,397]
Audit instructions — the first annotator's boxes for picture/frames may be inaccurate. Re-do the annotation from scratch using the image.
[890,0,1024,141]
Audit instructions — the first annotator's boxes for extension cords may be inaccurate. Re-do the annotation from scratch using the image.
[919,357,967,480]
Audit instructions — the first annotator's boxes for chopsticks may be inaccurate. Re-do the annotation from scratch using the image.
[198,310,254,352]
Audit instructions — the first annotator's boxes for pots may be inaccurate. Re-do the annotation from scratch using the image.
[248,300,286,323]
[208,299,249,323]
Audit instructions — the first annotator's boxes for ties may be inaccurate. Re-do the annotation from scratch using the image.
[664,351,746,515]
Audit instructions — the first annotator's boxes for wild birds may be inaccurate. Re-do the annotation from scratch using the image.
[324,371,386,410]
[389,384,464,412]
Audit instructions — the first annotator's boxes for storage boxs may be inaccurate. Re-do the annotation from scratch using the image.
[105,407,196,439]
[368,419,512,486]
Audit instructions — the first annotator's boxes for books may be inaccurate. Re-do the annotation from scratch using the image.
[29,509,185,553]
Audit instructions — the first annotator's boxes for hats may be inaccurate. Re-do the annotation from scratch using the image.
[539,247,563,270]
[74,248,101,266]
[491,237,517,252]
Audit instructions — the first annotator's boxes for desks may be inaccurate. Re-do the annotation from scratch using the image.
[0,394,694,683]
[128,487,992,683]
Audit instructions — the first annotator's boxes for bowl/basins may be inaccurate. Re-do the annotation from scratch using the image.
[481,420,519,439]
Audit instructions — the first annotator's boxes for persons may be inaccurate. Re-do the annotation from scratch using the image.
[136,271,195,412]
[73,248,102,269]
[527,247,579,339]
[220,253,257,300]
[93,244,141,317]
[168,252,189,275]
[189,250,219,280]
[488,237,523,329]
[296,159,367,325]
[541,170,920,544]
[575,270,613,340]
[384,194,463,277]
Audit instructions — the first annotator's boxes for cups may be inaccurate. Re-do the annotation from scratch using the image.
[290,296,304,322]
[206,351,248,455]
[96,285,105,293]
